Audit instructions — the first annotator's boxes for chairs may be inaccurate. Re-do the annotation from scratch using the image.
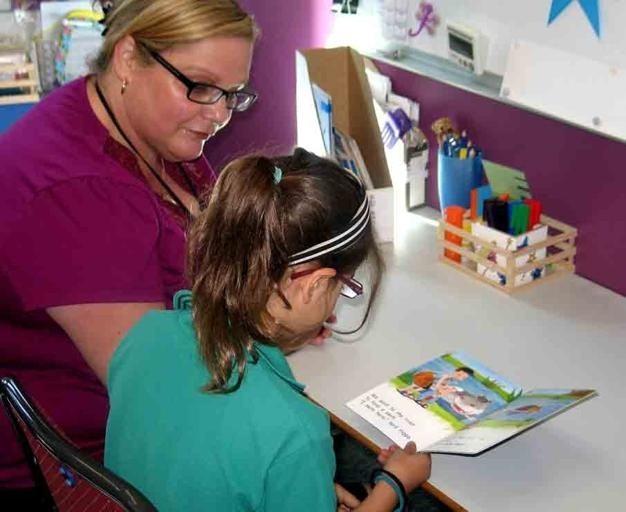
[0,377,158,512]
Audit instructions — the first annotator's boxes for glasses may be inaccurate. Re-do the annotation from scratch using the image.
[290,268,363,299]
[139,39,257,113]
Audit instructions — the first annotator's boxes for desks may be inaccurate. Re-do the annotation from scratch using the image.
[284,186,626,512]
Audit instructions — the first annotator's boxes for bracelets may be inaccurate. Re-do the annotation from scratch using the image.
[369,467,407,512]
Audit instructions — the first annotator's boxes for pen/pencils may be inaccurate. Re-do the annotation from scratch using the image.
[441,126,477,158]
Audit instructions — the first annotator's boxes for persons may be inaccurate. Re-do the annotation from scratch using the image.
[104,148,431,512]
[1,1,262,512]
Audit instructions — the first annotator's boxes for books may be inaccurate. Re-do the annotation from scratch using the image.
[345,349,595,455]
